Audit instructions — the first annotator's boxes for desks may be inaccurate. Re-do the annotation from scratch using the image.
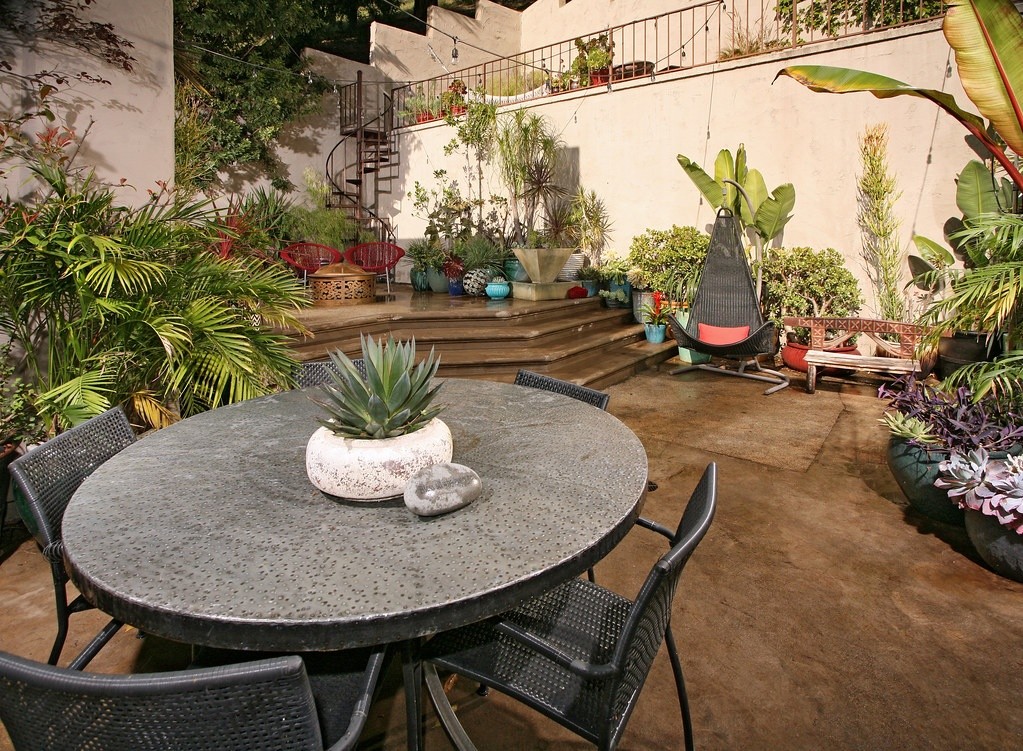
[63,376,648,654]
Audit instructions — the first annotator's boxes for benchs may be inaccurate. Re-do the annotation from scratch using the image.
[785,314,954,390]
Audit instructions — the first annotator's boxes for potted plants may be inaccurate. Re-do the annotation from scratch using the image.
[768,246,862,375]
[0,125,313,544]
[304,335,451,502]
[411,36,668,345]
[869,130,1023,583]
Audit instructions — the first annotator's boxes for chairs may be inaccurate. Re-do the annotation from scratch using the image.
[668,178,790,396]
[284,243,405,300]
[0,359,715,751]
